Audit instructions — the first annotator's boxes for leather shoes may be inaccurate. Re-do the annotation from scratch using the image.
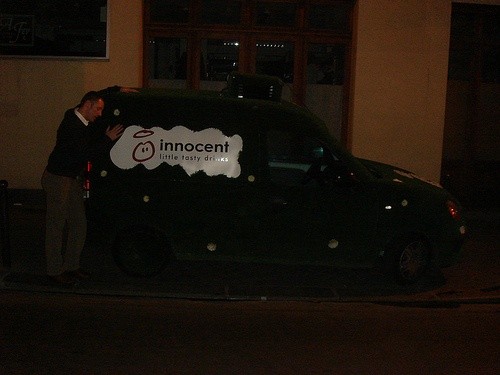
[66,269,91,281]
[45,275,78,284]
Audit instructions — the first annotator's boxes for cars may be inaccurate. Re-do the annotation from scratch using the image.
[79,82,466,294]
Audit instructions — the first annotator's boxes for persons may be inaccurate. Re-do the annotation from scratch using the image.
[41,85,141,285]
